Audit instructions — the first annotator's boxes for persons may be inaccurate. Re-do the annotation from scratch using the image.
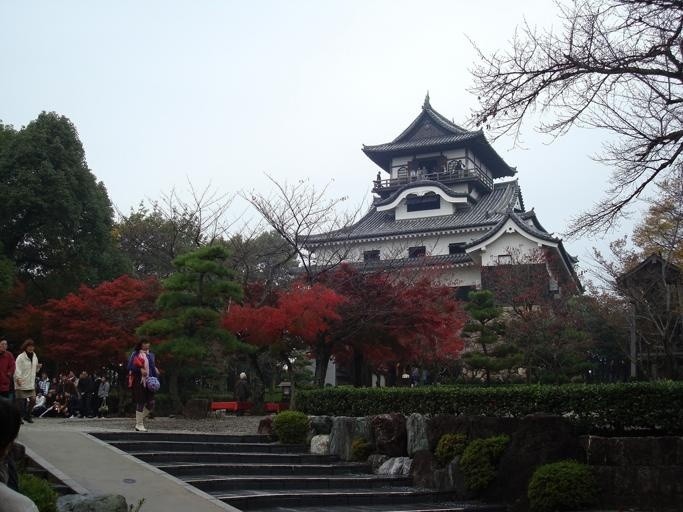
[0,338,16,403]
[0,397,39,512]
[128,337,160,431]
[377,171,381,188]
[408,158,465,182]
[12,338,39,423]
[233,372,250,417]
[31,370,111,419]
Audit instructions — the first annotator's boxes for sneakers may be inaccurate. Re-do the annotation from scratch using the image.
[20,414,34,425]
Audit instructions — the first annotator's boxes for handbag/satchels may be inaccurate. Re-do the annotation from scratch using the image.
[146,375,161,393]
[97,405,109,414]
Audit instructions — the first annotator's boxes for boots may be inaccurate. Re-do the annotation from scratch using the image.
[133,406,150,432]
